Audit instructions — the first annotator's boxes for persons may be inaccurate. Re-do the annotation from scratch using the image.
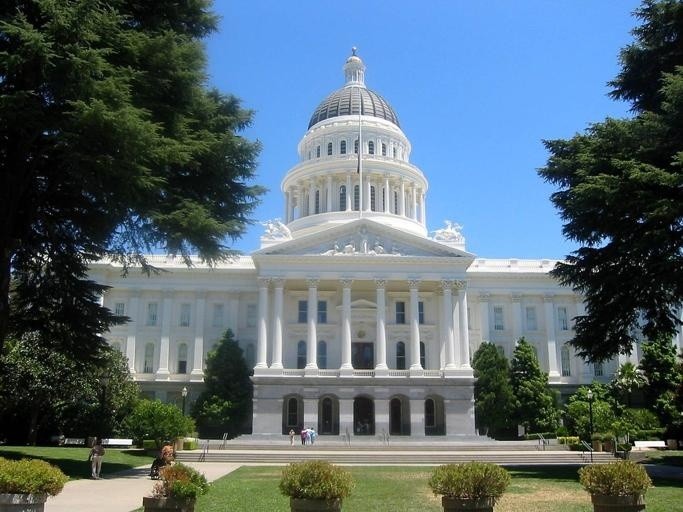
[88,441,104,479]
[300,427,316,446]
[288,428,294,446]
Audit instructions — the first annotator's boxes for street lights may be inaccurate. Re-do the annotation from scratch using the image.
[588,389,593,463]
[181,387,188,414]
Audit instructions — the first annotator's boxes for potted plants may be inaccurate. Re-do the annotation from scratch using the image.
[143,461,209,512]
[0,457,70,512]
[278,460,357,512]
[428,460,513,512]
[577,460,653,512]
